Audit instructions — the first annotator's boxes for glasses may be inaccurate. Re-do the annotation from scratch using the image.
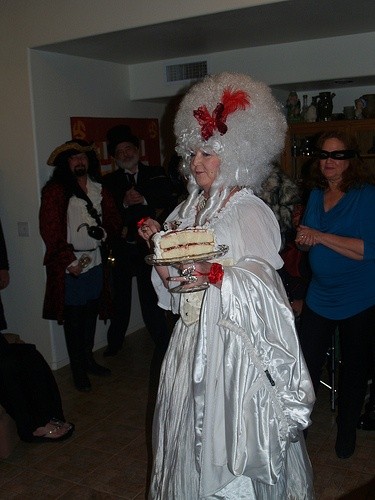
[319,150,355,160]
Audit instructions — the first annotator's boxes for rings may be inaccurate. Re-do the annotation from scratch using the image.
[300,234,304,239]
[141,224,149,234]
[182,263,196,277]
[187,275,198,283]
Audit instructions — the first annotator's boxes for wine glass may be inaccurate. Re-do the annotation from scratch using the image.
[78,253,92,268]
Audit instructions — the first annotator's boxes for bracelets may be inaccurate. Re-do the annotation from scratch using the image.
[195,262,224,285]
[137,216,150,228]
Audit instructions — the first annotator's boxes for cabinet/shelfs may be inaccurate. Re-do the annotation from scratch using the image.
[279,118,375,192]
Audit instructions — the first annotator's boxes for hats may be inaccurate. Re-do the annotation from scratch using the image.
[47,139,102,166]
[106,125,141,155]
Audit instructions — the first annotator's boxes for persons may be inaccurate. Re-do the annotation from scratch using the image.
[92,124,178,357]
[39,138,123,391]
[0,331,75,441]
[285,92,301,119]
[306,105,317,123]
[355,99,368,120]
[0,218,9,331]
[294,130,375,459]
[137,124,316,500]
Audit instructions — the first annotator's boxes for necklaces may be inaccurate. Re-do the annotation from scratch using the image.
[195,185,238,213]
[324,190,343,210]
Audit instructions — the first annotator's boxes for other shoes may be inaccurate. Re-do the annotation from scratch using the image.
[334,429,357,460]
[33,419,74,441]
